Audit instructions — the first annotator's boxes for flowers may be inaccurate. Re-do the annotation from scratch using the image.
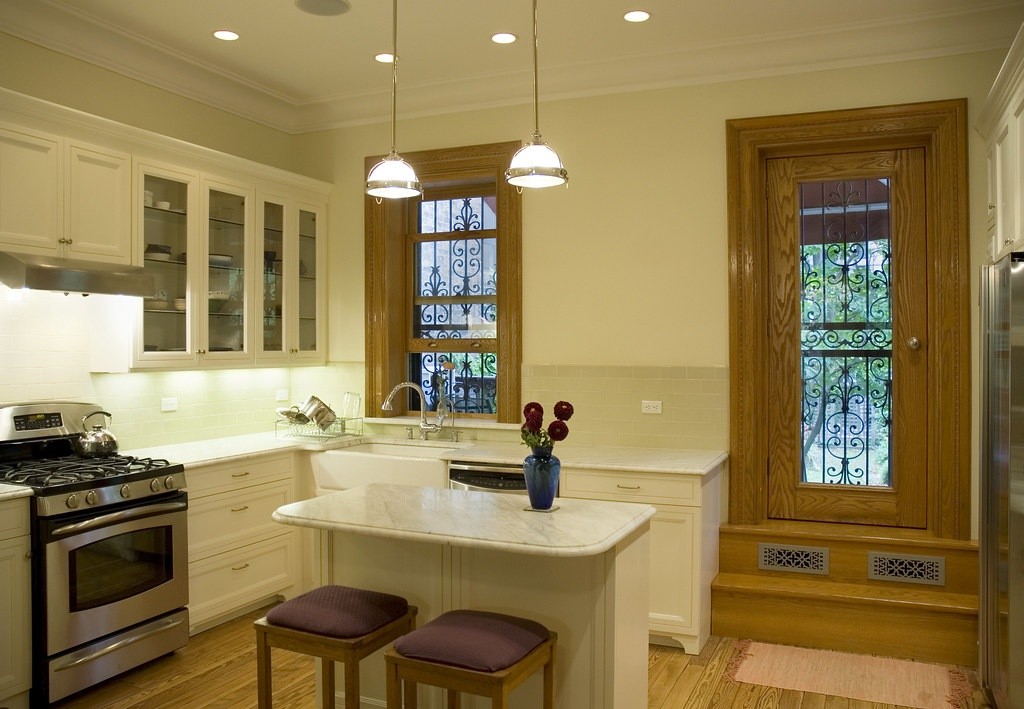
[517,400,575,457]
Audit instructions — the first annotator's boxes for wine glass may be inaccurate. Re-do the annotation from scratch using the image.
[264,251,276,272]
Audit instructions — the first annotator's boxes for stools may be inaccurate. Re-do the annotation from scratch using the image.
[382,608,558,709]
[253,584,419,709]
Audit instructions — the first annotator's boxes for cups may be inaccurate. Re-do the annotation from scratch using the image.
[264,307,273,315]
[155,201,170,210]
[144,190,153,207]
[343,392,361,429]
[276,305,281,315]
[273,259,282,273]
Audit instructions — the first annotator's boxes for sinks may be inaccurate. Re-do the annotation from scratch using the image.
[317,443,459,489]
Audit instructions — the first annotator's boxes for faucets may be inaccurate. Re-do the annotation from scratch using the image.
[380,382,445,440]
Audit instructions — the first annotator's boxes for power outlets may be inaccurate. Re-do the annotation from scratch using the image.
[641,400,664,414]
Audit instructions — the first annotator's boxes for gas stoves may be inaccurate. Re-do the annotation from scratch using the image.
[0,398,188,516]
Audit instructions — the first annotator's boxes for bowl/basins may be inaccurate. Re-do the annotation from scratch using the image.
[174,298,186,311]
[214,207,232,219]
[144,301,167,309]
[209,299,228,313]
[144,344,158,351]
[145,244,171,254]
[278,412,309,424]
[144,253,171,260]
[208,291,229,299]
[290,395,336,431]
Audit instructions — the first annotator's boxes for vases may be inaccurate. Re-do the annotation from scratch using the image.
[521,452,562,510]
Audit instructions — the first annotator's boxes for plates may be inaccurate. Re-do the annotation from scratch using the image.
[182,252,233,266]
[209,347,233,351]
[160,347,186,350]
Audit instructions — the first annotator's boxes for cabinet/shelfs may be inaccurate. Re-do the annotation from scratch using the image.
[184,451,303,637]
[270,482,658,709]
[560,463,724,657]
[0,86,334,374]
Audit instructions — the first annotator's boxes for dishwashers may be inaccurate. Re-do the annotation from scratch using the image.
[446,461,560,498]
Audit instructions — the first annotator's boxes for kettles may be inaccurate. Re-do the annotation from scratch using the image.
[77,411,120,457]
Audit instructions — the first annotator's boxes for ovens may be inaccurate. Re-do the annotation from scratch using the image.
[30,483,190,709]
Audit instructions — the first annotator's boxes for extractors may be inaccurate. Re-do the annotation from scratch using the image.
[0,251,153,296]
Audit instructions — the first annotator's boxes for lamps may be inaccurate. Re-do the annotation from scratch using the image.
[504,0,570,190]
[365,0,422,200]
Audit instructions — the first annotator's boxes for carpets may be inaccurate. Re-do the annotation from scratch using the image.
[720,638,974,709]
